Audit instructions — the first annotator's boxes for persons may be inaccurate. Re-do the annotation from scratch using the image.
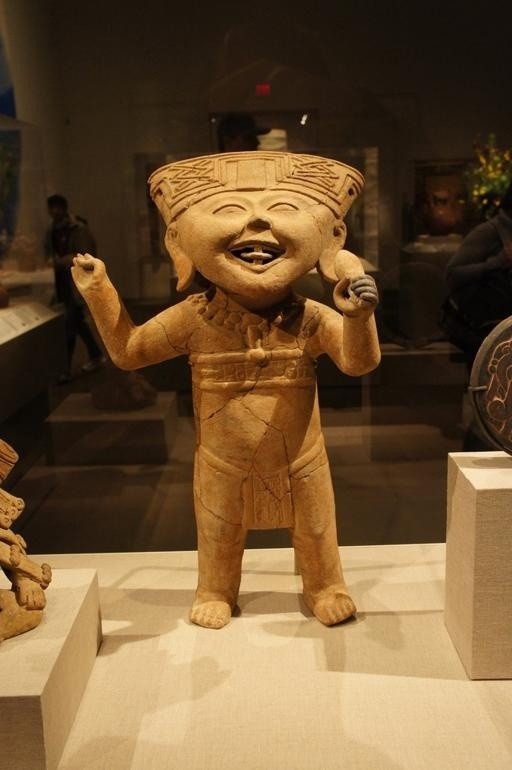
[47,194,108,370]
[435,186,512,355]
[69,150,382,628]
